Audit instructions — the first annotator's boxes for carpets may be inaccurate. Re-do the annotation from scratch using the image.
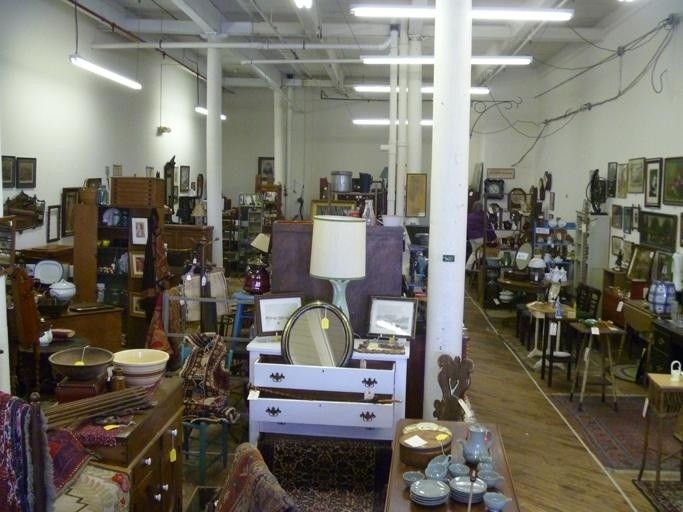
[256,434,391,512]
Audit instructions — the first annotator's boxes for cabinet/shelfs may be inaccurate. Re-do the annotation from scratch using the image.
[602,268,683,383]
[480,193,576,309]
[244,335,411,449]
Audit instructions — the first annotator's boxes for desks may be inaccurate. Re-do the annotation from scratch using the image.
[495,276,572,302]
[383,418,520,511]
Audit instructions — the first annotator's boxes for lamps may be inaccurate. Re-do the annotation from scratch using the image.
[68,3,142,91]
[309,214,367,321]
[527,249,546,285]
[349,5,574,127]
[193,52,227,121]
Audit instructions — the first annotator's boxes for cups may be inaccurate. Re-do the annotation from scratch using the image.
[477,469,505,489]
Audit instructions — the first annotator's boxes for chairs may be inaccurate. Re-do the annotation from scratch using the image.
[468,243,500,293]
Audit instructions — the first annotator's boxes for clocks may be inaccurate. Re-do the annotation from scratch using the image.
[483,179,505,199]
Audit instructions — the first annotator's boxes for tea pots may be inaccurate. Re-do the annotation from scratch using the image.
[456,424,494,463]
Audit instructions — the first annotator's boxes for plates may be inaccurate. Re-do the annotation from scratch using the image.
[409,475,487,506]
[34,260,63,285]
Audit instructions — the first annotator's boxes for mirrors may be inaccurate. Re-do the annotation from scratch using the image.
[280,300,354,367]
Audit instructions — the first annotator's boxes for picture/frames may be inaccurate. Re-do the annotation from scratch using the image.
[46,155,203,243]
[607,157,683,282]
[15,157,36,189]
[308,199,357,222]
[257,157,274,181]
[253,292,419,340]
[1,156,15,188]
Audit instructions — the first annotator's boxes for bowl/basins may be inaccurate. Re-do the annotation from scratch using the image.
[403,454,470,489]
[483,492,507,512]
[47,345,115,380]
[112,347,171,389]
